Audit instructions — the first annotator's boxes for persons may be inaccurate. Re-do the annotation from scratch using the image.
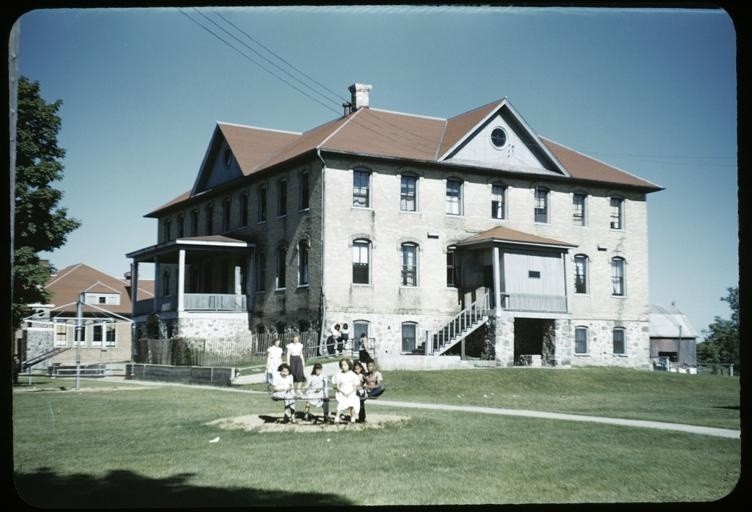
[272,363,299,425]
[302,362,330,422]
[264,338,286,395]
[353,362,368,424]
[326,323,342,355]
[357,332,375,372]
[332,357,364,425]
[365,359,386,399]
[337,323,350,354]
[287,332,308,394]
